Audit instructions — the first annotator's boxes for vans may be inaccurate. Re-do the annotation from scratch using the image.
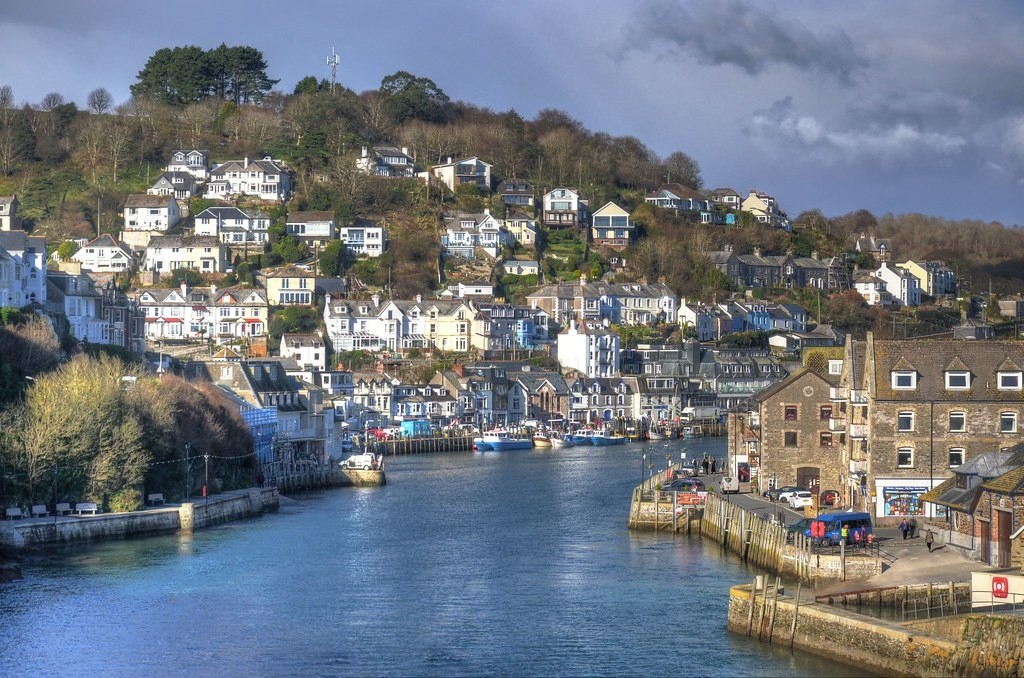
[718,477,739,495]
[802,512,873,548]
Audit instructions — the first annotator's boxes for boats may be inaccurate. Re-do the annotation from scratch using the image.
[473,430,532,451]
[646,417,704,440]
[533,422,627,449]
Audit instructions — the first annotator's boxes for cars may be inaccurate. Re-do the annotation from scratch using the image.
[660,464,705,496]
[366,429,391,441]
[786,517,817,540]
[763,485,814,511]
[820,490,840,505]
[342,441,352,453]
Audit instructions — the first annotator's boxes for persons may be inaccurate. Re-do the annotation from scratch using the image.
[907,516,918,539]
[860,526,867,548]
[924,529,934,552]
[255,471,265,488]
[897,518,910,540]
[867,533,876,550]
[840,524,850,550]
[854,530,860,548]
[691,455,726,476]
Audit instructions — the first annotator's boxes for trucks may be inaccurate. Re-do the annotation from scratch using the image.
[680,406,722,425]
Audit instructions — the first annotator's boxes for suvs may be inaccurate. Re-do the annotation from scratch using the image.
[338,454,376,471]
